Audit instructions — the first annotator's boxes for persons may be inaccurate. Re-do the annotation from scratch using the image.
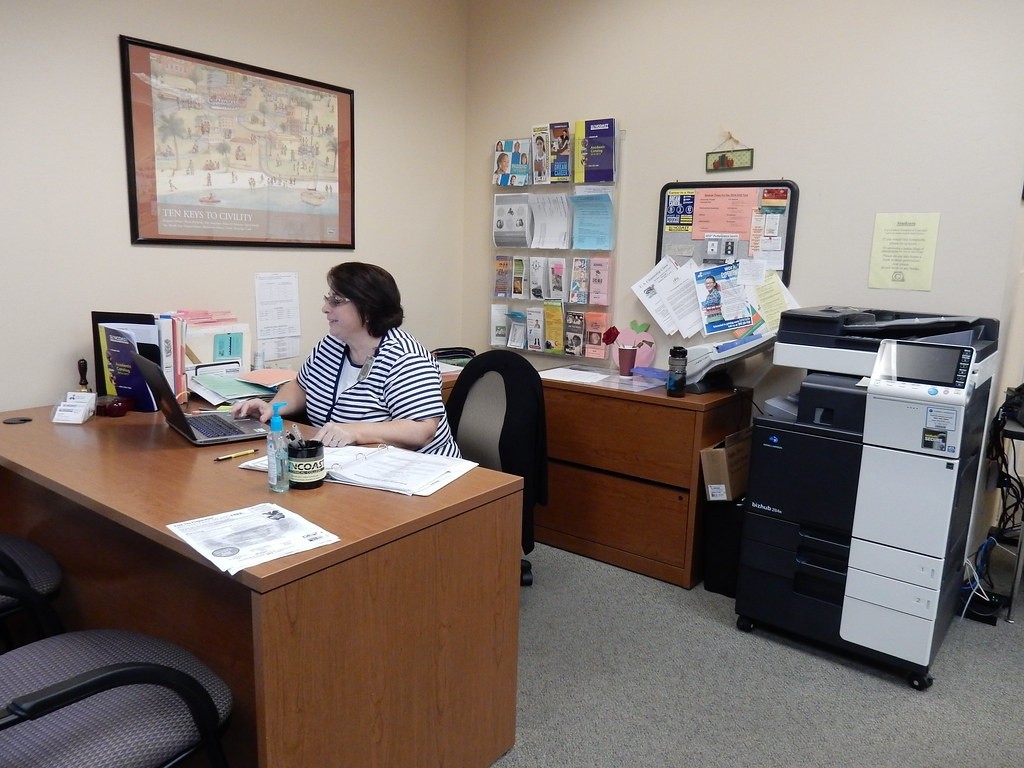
[493,129,570,186]
[701,276,725,322]
[231,262,463,460]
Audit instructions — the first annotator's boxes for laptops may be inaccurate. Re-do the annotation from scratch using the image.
[130,351,271,445]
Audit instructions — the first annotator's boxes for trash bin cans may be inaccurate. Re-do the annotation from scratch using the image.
[701,498,744,597]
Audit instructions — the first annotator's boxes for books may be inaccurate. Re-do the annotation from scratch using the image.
[89,309,301,412]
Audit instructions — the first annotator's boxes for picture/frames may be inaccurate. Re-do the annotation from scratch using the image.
[118,34,356,251]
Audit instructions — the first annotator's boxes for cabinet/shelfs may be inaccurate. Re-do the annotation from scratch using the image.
[487,129,626,368]
[536,364,754,590]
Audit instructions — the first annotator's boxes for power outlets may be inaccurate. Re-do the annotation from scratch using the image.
[987,454,1006,493]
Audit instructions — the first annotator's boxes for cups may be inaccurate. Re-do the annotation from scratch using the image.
[288,440,326,490]
[618,346,637,376]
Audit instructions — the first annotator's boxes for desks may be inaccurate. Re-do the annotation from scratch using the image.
[0,363,524,768]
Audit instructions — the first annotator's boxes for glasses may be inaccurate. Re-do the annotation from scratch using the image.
[323,287,351,308]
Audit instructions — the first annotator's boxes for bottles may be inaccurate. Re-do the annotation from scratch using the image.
[666,345,687,398]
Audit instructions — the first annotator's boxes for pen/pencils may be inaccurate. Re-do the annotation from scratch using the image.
[192,410,233,415]
[212,449,259,461]
[285,430,300,447]
[291,423,305,447]
[199,407,214,411]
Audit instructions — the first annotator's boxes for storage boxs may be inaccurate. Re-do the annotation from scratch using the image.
[700,427,753,501]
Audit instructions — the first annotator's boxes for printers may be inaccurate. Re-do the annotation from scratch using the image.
[734,303,1001,690]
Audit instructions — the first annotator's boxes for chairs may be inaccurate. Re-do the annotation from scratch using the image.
[0,627,234,768]
[445,350,548,586]
[0,534,62,654]
